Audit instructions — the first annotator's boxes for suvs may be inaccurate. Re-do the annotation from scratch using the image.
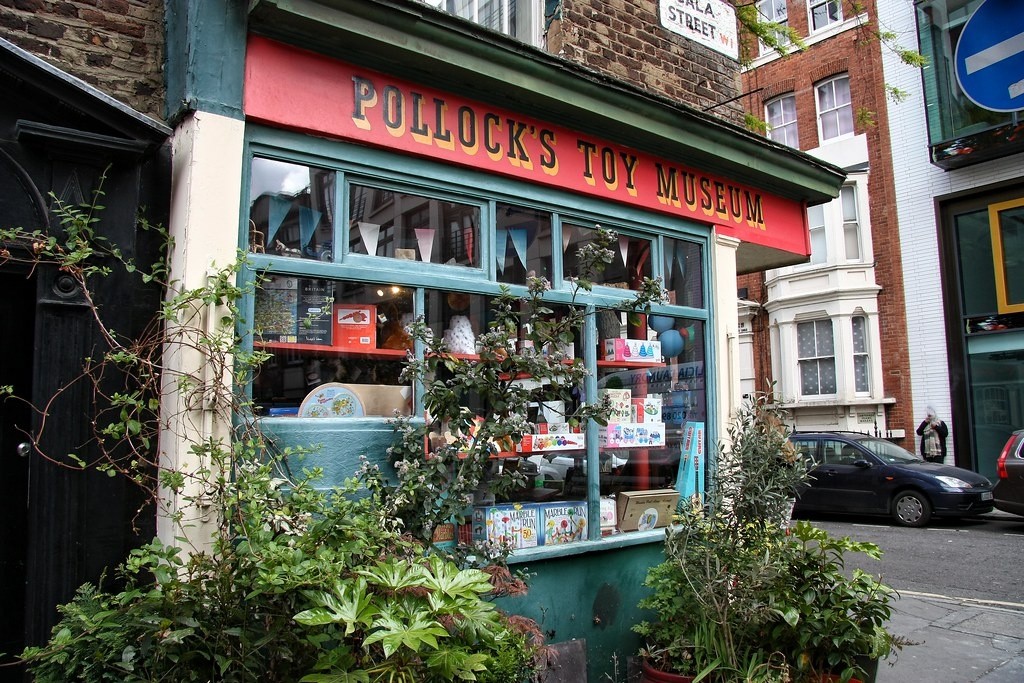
[782,430,993,528]
[987,430,1024,517]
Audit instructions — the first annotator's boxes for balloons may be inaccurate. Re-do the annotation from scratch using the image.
[657,330,685,357]
[648,314,675,333]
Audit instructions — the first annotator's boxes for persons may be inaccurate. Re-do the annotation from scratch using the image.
[917,405,949,464]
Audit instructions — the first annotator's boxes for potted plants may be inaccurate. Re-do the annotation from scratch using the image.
[629,376,926,683]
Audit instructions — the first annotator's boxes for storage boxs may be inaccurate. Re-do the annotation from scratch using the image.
[539,500,589,546]
[440,333,665,453]
[617,489,680,530]
[255,277,377,349]
[471,503,539,551]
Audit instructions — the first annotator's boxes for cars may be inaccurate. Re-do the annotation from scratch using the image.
[611,430,686,494]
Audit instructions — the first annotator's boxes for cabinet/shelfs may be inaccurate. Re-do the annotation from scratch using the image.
[253,342,667,458]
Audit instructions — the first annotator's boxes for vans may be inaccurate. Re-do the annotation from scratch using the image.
[539,361,705,495]
